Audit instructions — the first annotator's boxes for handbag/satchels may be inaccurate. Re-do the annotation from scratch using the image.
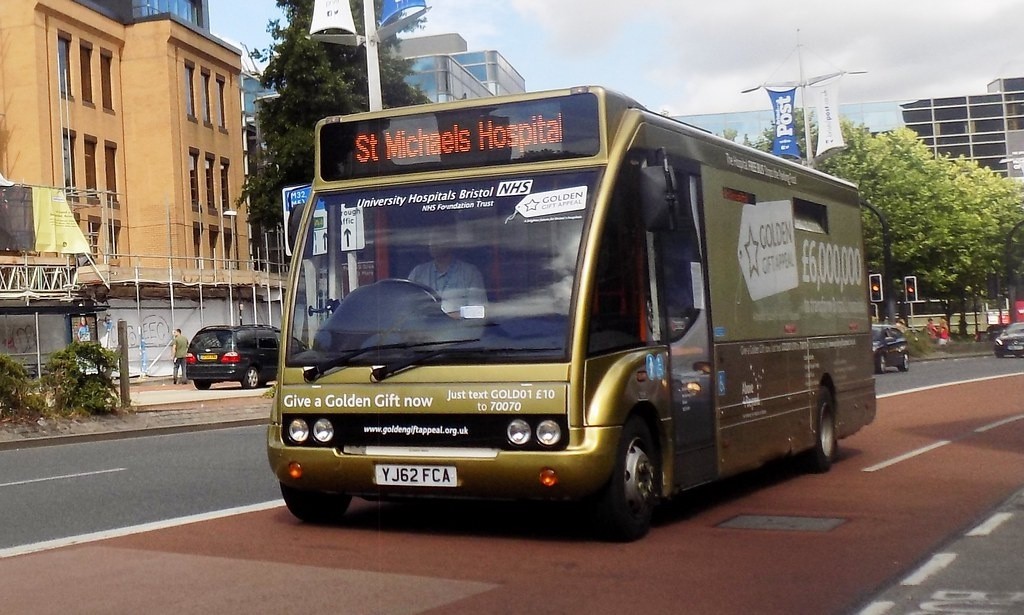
[938,338,947,345]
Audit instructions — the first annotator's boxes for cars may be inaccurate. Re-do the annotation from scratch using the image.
[872,325,910,374]
[993,322,1024,358]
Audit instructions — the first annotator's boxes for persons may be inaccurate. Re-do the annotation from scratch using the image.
[172,329,189,384]
[77,317,91,342]
[895,316,949,345]
[407,216,490,319]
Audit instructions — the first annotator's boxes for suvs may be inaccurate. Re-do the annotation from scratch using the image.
[186,324,312,390]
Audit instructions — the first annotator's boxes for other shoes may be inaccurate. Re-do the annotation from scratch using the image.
[174,379,177,384]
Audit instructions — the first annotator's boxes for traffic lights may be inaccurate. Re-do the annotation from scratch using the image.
[904,276,917,303]
[869,274,884,302]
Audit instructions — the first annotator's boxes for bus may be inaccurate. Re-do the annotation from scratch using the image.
[266,85,877,543]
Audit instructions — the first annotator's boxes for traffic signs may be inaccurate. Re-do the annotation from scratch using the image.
[281,184,367,257]
